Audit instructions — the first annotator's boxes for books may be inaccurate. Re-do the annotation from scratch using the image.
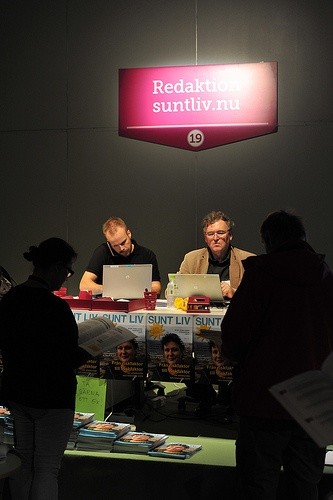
[266,351,333,449]
[0,405,14,446]
[77,316,137,355]
[65,411,202,460]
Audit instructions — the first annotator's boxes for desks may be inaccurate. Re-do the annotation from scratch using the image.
[64,436,333,474]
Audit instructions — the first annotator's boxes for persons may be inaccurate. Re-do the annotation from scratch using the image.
[151,334,191,383]
[150,444,190,455]
[115,433,155,445]
[219,210,333,500]
[0,237,98,500]
[73,413,86,427]
[101,338,143,379]
[82,422,118,432]
[78,215,161,300]
[165,212,257,301]
[198,340,238,389]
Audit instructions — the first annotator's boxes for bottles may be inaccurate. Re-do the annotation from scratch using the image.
[167,274,178,310]
[144,291,157,310]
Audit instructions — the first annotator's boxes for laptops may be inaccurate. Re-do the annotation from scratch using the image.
[93,264,152,299]
[168,273,230,309]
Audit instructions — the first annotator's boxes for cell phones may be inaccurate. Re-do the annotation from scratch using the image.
[106,241,119,256]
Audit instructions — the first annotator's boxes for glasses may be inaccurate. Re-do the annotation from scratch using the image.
[65,265,76,279]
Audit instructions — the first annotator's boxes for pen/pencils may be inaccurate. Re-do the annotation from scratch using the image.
[144,287,157,310]
